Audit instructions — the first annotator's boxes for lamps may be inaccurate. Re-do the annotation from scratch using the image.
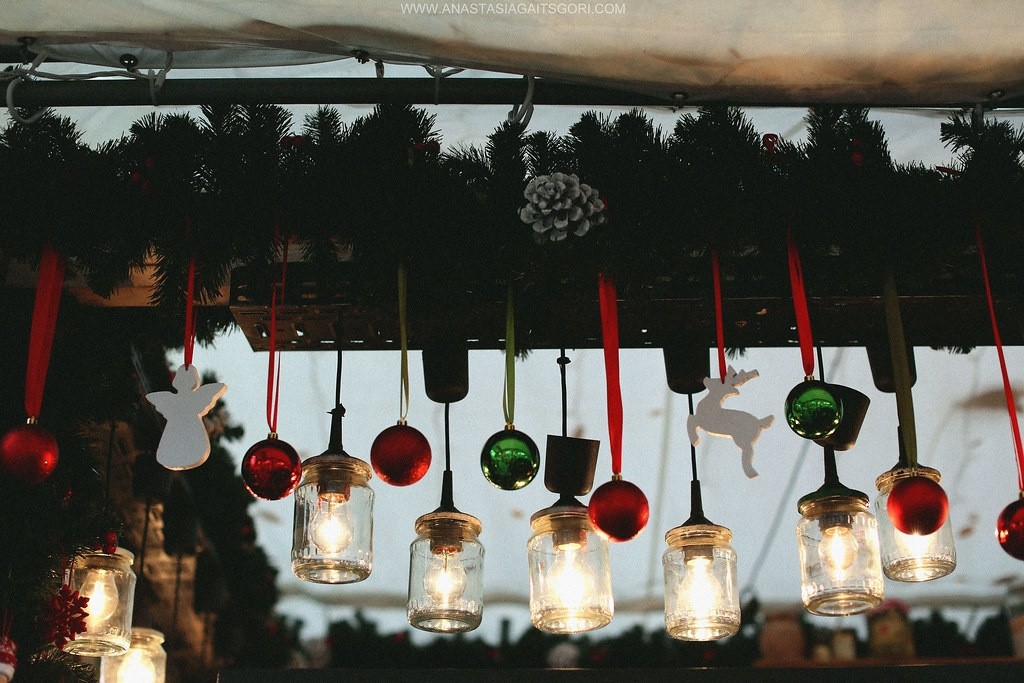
[527,348,614,636]
[405,400,485,634]
[98,478,168,683]
[796,347,885,617]
[661,391,741,643]
[875,423,956,583]
[291,318,374,585]
[62,417,137,656]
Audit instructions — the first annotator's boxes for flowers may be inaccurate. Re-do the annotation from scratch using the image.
[517,171,608,243]
